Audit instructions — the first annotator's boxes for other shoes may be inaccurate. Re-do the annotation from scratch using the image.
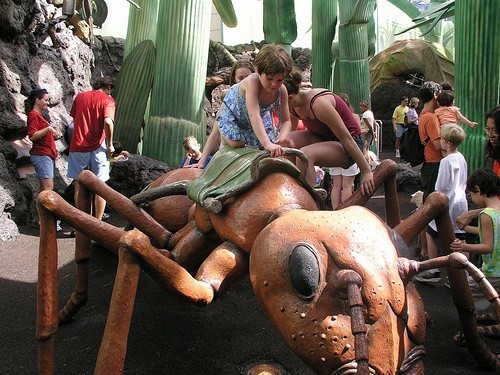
[419,256,428,261]
[415,248,421,257]
[102,213,110,220]
[396,153,400,157]
[57,220,62,233]
[415,268,441,282]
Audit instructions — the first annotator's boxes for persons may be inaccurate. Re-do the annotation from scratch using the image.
[440,83,453,91]
[329,93,370,210]
[25,88,63,232]
[216,42,295,158]
[407,97,418,131]
[434,90,478,158]
[414,81,442,261]
[456,107,500,230]
[414,123,468,288]
[66,76,115,237]
[184,59,280,169]
[392,96,409,158]
[314,166,324,183]
[450,170,500,343]
[182,135,212,168]
[103,141,129,219]
[282,72,375,197]
[359,100,377,150]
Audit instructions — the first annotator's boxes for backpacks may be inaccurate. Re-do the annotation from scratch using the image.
[399,111,431,167]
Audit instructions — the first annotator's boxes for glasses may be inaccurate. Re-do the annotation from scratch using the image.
[484,127,499,136]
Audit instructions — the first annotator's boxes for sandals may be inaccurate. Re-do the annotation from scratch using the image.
[476,313,498,323]
[479,325,499,338]
